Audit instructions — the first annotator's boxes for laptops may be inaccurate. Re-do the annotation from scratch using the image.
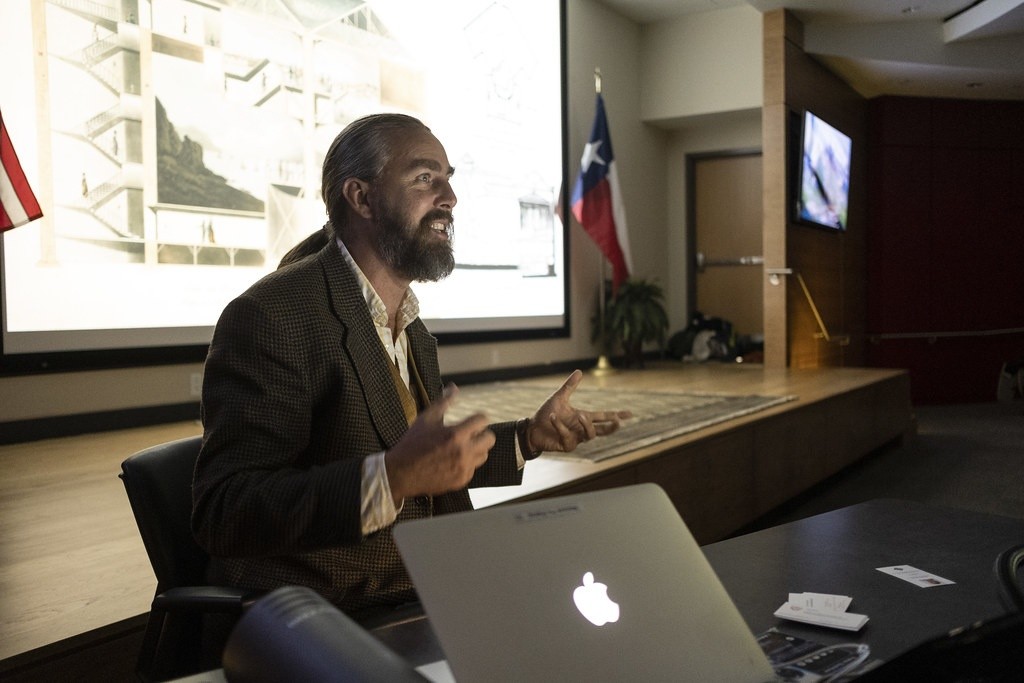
[393,482,779,683]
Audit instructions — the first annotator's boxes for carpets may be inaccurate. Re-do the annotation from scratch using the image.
[444,380,801,462]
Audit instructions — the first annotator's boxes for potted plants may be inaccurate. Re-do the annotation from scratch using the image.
[590,277,670,369]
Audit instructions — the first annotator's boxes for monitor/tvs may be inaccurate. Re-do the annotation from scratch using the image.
[791,110,854,234]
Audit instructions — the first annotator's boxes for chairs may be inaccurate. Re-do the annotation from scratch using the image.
[117,437,242,683]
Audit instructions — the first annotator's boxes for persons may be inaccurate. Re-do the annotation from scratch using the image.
[187,111,637,623]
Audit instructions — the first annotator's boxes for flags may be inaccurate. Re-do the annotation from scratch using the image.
[0,111,46,235]
[567,72,634,302]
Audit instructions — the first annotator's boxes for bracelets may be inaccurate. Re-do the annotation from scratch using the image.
[517,418,545,461]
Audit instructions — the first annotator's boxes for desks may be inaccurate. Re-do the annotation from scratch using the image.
[366,499,1024,683]
